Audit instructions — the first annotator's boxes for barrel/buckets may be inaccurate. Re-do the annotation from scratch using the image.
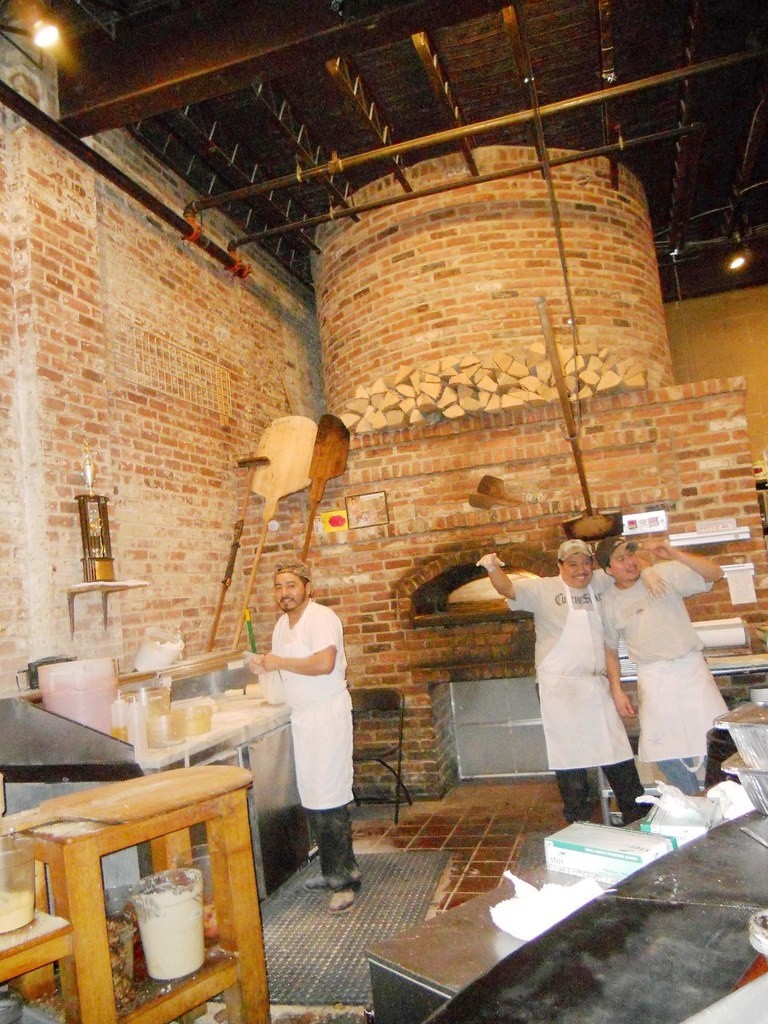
[134,625,185,675]
[37,656,118,738]
[0,836,35,936]
[106,844,220,1010]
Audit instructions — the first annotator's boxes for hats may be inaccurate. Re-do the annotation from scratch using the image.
[273,561,312,581]
[595,538,638,572]
[558,539,592,561]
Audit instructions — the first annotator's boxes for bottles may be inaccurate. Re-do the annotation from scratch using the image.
[112,690,128,743]
[126,694,149,752]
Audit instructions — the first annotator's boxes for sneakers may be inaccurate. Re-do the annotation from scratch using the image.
[304,874,332,892]
[329,882,359,915]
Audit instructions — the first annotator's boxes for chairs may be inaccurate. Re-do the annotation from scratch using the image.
[348,687,414,826]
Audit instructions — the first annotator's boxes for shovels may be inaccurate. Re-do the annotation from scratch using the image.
[535,294,624,542]
[477,474,524,504]
[300,414,350,564]
[229,415,319,650]
[469,493,518,510]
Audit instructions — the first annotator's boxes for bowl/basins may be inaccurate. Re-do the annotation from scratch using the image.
[124,686,212,750]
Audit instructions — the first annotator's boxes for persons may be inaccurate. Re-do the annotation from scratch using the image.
[594,538,731,796]
[250,557,362,915]
[475,539,669,829]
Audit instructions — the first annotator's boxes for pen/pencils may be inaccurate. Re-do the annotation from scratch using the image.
[739,825,768,848]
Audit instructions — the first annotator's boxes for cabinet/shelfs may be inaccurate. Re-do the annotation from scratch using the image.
[19,764,274,1023]
